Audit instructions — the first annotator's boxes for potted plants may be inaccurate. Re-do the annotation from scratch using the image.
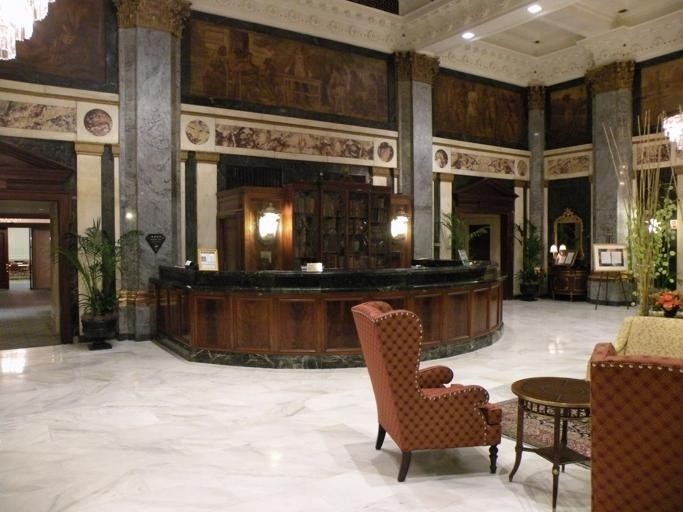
[36,217,145,350]
[512,219,547,301]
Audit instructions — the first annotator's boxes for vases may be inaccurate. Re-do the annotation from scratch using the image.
[663,307,680,318]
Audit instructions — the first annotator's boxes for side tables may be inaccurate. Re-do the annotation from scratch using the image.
[508,376,592,511]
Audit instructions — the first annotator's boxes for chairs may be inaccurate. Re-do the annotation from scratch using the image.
[585,314,682,511]
[348,297,503,482]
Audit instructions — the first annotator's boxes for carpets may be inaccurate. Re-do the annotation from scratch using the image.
[482,397,595,470]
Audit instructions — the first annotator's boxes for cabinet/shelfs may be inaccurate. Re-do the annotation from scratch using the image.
[551,267,586,303]
[283,181,390,271]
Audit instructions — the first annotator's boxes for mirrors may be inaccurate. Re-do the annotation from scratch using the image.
[554,207,584,259]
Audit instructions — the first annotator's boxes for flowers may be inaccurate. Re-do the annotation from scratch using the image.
[647,290,682,311]
